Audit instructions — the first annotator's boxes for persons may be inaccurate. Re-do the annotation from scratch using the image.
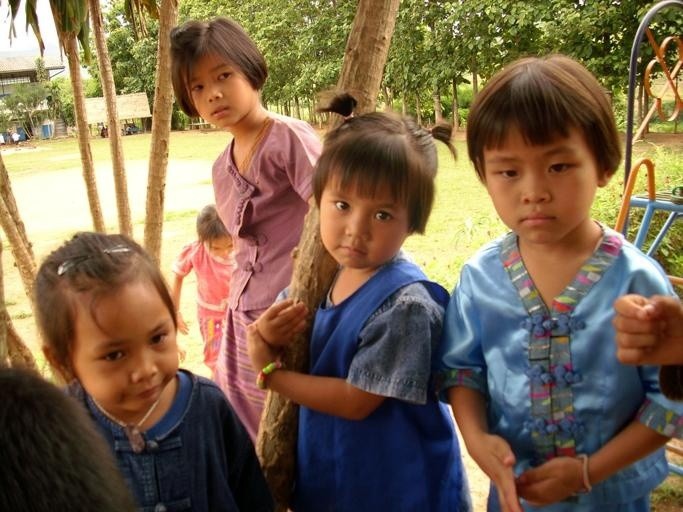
[434,49,681,512]
[0,366,138,512]
[246,88,475,512]
[126,126,131,132]
[101,126,108,138]
[12,131,20,144]
[613,292,683,401]
[169,18,325,452]
[608,283,683,408]
[34,229,275,512]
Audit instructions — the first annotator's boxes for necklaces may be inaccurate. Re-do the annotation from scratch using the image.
[91,394,159,455]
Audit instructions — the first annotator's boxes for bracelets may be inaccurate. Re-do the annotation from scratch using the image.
[575,449,598,497]
[576,452,593,496]
